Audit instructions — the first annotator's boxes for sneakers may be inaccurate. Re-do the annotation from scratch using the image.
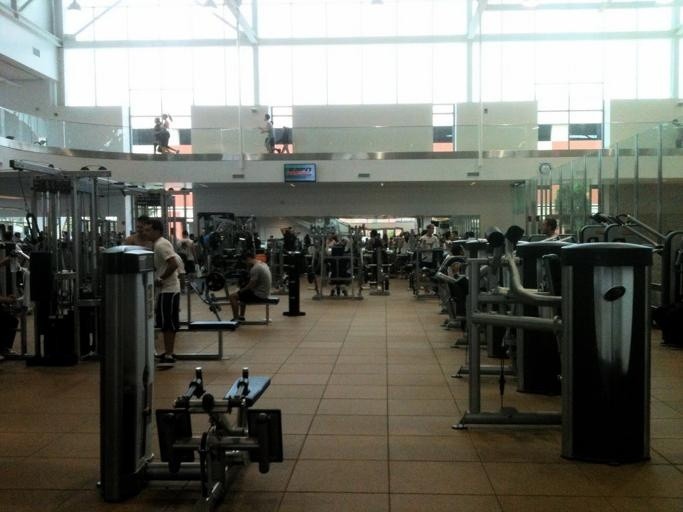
[156,353,176,368]
[237,317,246,322]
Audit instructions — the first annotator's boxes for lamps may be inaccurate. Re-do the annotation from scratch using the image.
[539,163,552,175]
[204,0,245,36]
[18,0,79,10]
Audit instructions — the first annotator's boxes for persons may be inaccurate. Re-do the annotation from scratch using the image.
[259,114,274,153]
[326,236,336,249]
[12,232,22,242]
[229,253,272,322]
[154,118,171,155]
[366,217,558,279]
[122,218,196,362]
[161,115,179,155]
[270,121,281,153]
[281,127,290,154]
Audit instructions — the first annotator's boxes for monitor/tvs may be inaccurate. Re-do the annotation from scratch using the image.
[284,164,316,182]
